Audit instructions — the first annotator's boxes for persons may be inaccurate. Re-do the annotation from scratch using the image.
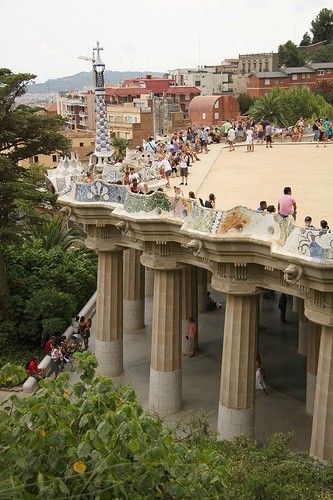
[45,315,92,380]
[110,117,333,234]
[278,187,297,221]
[25,356,45,381]
[255,352,266,390]
[84,171,94,183]
[278,293,288,322]
[186,317,197,357]
[208,291,221,310]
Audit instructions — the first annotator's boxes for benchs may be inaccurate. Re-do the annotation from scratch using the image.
[74,126,333,262]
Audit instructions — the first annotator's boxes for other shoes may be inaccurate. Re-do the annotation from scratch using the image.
[180,183,184,184]
[185,183,187,185]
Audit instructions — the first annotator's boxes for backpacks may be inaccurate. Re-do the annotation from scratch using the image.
[25,360,36,375]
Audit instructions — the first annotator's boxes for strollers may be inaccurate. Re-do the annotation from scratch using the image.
[66,333,83,354]
